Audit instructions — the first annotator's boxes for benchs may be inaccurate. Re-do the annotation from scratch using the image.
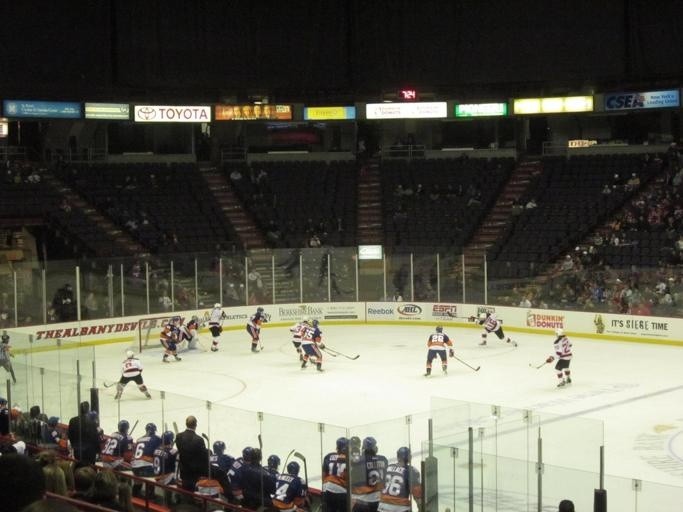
[0,430,255,511]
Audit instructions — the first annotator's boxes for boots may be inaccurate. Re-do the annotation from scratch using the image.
[174,353,181,360]
[441,364,448,375]
[163,354,170,361]
[115,391,122,398]
[424,368,431,376]
[144,391,151,398]
[302,360,308,368]
[566,376,572,383]
[317,362,323,371]
[513,341,518,347]
[478,340,487,345]
[211,346,217,352]
[251,343,259,351]
[557,378,566,387]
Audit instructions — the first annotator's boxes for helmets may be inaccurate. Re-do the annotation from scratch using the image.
[397,447,411,462]
[118,420,129,433]
[161,431,174,444]
[555,328,564,336]
[287,462,299,474]
[256,307,265,314]
[479,313,487,322]
[126,350,133,358]
[254,448,262,461]
[350,437,361,449]
[436,325,442,332]
[88,411,99,426]
[336,437,349,450]
[213,441,226,453]
[268,455,280,469]
[362,437,377,452]
[312,320,318,327]
[243,447,255,462]
[146,423,156,435]
[214,302,221,308]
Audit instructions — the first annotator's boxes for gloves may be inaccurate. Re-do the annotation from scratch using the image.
[479,320,484,325]
[449,349,454,357]
[320,343,325,349]
[547,355,554,363]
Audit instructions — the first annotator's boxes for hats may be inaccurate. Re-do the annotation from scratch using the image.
[47,417,59,426]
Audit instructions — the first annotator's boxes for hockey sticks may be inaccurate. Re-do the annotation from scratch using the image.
[325,347,360,360]
[104,382,116,388]
[322,348,337,357]
[529,363,548,370]
[447,311,468,319]
[453,355,481,372]
[258,433,263,450]
[260,346,264,350]
[284,449,294,476]
[294,452,308,486]
[202,433,209,441]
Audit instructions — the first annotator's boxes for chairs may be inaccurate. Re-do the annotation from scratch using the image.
[0,149,682,331]
